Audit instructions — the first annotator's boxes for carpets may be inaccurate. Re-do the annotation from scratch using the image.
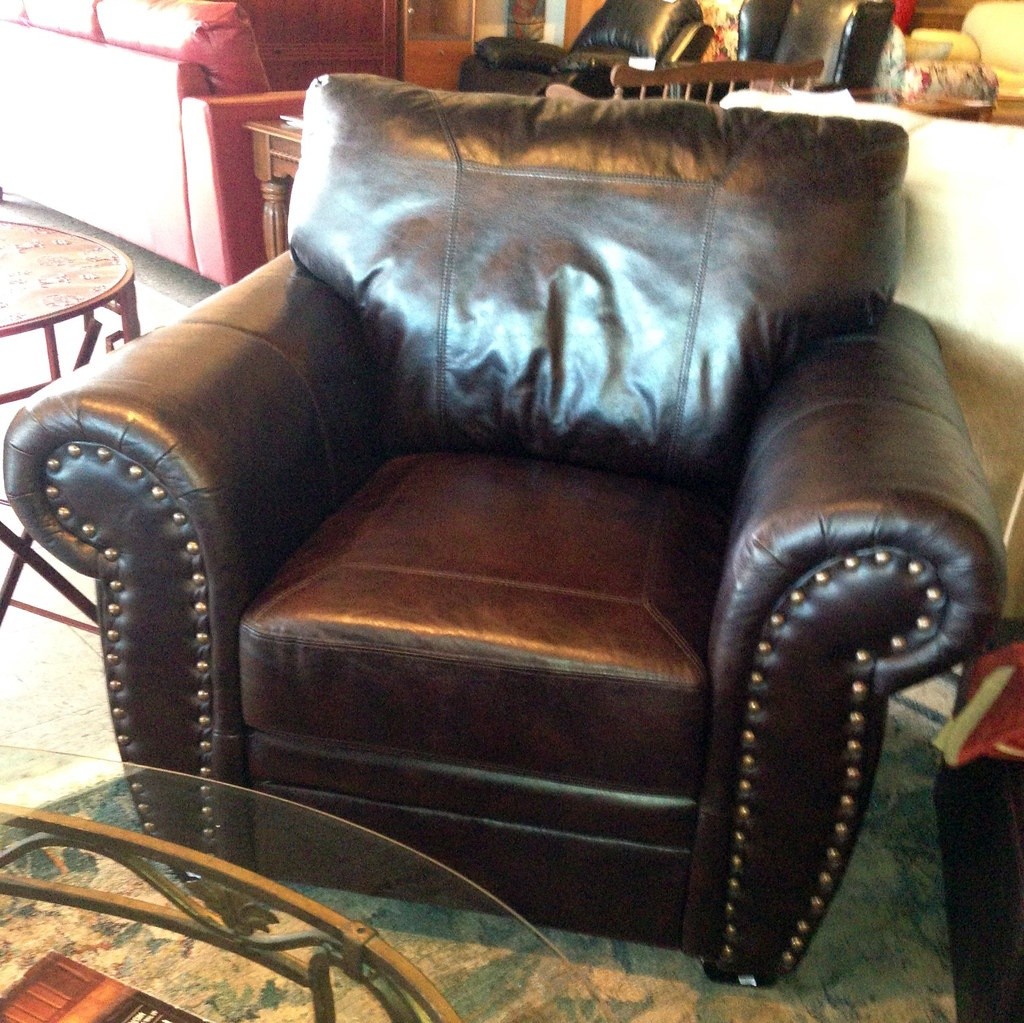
[1,688,964,1022]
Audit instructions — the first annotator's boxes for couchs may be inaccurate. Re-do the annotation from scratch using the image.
[458,0,715,99]
[3,73,1008,988]
[0,0,308,288]
[656,0,895,105]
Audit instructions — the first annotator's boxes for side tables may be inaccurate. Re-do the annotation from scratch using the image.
[244,114,303,262]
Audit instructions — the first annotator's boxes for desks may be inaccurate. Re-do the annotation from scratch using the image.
[0,219,141,637]
[0,745,618,1023]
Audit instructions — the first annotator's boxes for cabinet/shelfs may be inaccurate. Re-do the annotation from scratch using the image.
[396,0,477,92]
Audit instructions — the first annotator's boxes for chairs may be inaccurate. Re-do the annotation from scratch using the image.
[544,59,825,105]
